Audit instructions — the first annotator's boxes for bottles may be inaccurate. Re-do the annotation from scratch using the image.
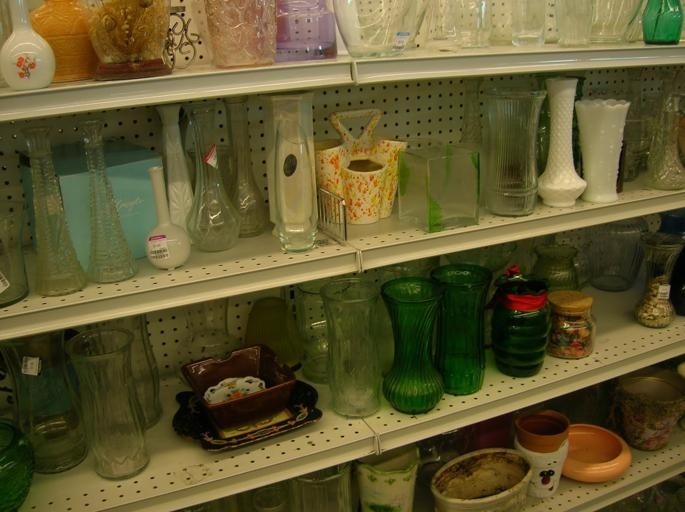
[0,0,56,91]
[144,165,191,269]
[546,290,595,358]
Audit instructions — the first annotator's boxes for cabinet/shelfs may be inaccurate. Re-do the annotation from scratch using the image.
[0,0,685,512]
